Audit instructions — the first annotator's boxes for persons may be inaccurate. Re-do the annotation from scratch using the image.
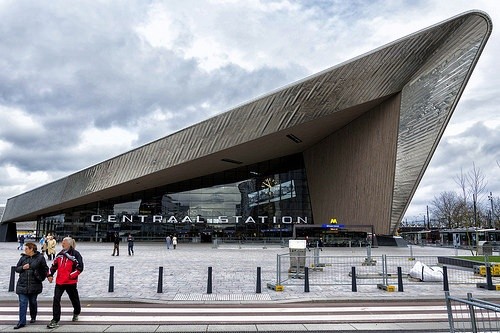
[39,234,57,260]
[13,243,48,329]
[111,233,119,256]
[46,237,83,328]
[172,236,177,249]
[304,236,312,252]
[166,236,171,249]
[318,237,324,251]
[127,234,135,256]
[17,235,24,250]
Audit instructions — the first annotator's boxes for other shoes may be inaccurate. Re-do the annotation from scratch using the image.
[14,323,25,328]
[72,315,78,321]
[47,319,59,327]
[30,318,36,323]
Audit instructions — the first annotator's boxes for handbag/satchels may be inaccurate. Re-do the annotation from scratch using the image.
[34,268,47,281]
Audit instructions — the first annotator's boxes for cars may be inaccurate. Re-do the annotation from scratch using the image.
[19,234,36,239]
